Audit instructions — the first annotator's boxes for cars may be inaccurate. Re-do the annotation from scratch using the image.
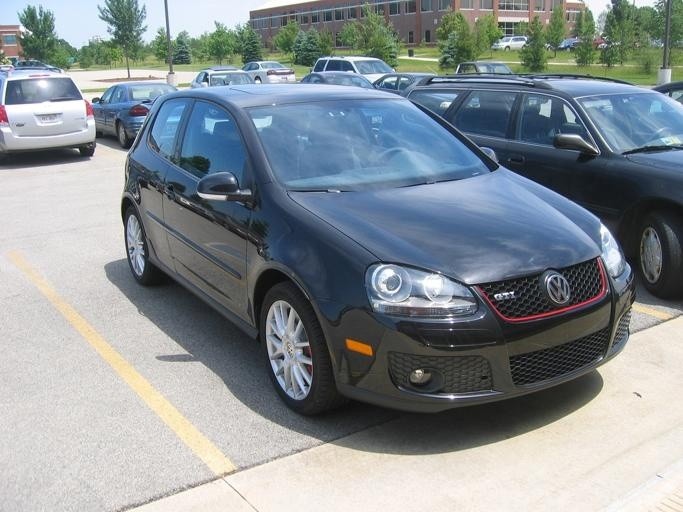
[0,55,97,163]
[121,84,636,405]
[240,61,296,83]
[375,72,683,300]
[402,63,682,134]
[492,34,609,53]
[299,57,435,94]
[92,82,178,149]
[191,66,256,88]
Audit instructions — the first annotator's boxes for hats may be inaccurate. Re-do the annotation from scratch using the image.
[92,82,178,149]
[0,55,97,163]
[240,61,296,83]
[492,34,609,53]
[299,57,435,94]
[191,66,256,88]
[121,84,636,405]
